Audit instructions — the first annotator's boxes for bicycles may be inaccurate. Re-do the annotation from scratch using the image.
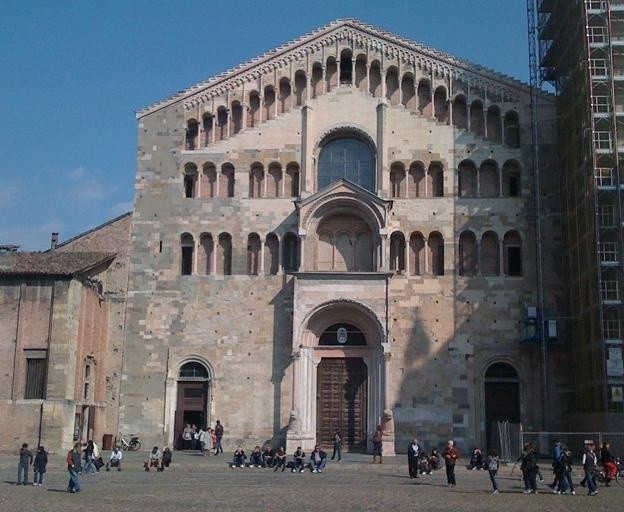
[113,432,141,451]
[592,457,624,490]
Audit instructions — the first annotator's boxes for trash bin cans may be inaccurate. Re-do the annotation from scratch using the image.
[102,434,113,450]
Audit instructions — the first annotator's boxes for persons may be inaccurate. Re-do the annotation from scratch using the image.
[307,444,327,473]
[64,417,224,492]
[260,445,274,469]
[272,446,287,469]
[370,424,382,463]
[16,442,33,485]
[32,445,47,486]
[407,428,620,497]
[329,428,342,462]
[291,445,306,473]
[230,443,246,468]
[247,445,262,469]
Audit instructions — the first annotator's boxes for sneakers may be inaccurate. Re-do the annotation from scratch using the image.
[330,459,335,462]
[523,482,611,496]
[232,464,322,473]
[80,468,121,475]
[410,472,433,479]
[67,490,75,493]
[76,489,81,492]
[145,467,149,471]
[448,484,452,487]
[480,469,483,470]
[453,484,456,487]
[16,482,43,487]
[471,468,477,470]
[493,490,499,494]
[157,469,161,472]
[338,460,342,462]
